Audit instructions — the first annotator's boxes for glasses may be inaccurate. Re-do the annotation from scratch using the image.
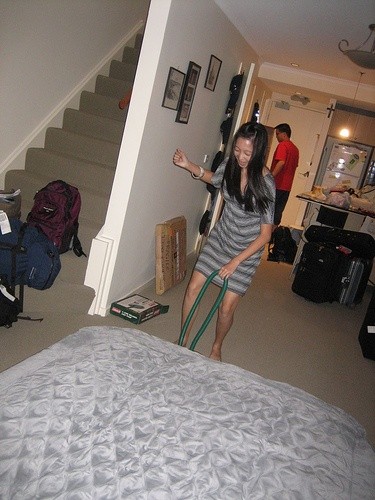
[276,131,283,135]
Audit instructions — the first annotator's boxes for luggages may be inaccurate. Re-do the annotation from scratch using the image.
[291,243,373,305]
[359,292,375,360]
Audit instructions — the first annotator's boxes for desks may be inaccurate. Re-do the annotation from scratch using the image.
[287,193,375,278]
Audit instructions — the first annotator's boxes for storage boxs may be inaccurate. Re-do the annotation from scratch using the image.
[110,293,169,326]
[155,216,186,295]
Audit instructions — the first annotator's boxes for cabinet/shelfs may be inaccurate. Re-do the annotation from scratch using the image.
[328,109,375,147]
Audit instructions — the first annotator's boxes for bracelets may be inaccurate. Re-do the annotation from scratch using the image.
[191,166,204,180]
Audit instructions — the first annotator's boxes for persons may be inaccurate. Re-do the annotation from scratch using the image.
[269,123,299,233]
[173,120,277,362]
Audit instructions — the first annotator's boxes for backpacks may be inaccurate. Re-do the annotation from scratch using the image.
[26,180,88,258]
[266,226,298,265]
[302,224,375,260]
[0,189,62,328]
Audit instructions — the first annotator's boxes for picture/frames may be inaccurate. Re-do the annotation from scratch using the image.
[204,55,223,92]
[162,66,186,111]
[174,60,202,124]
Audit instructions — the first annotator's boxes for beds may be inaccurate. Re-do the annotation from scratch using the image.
[0,327,375,499]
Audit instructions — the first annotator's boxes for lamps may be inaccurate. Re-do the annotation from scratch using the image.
[338,23,375,71]
